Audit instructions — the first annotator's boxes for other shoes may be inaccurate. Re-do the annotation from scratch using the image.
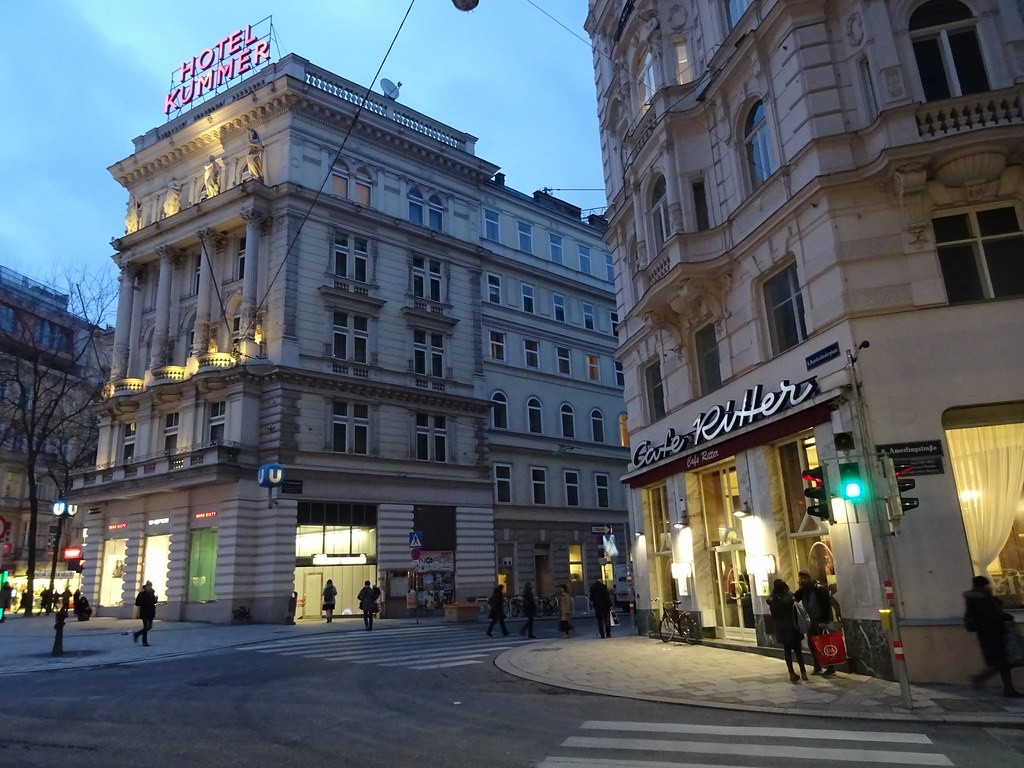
[327,617,332,623]
[142,642,150,646]
[504,632,511,636]
[528,635,537,638]
[971,674,983,690]
[606,634,611,638]
[1004,688,1024,699]
[520,630,525,636]
[601,634,605,638]
[134,633,138,643]
[801,671,809,681]
[790,673,800,683]
[365,626,372,631]
[823,668,835,675]
[487,631,492,636]
[811,665,821,674]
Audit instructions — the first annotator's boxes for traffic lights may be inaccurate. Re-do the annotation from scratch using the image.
[833,432,855,451]
[800,465,829,522]
[839,462,862,501]
[896,460,920,511]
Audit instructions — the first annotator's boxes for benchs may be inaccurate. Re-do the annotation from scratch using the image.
[444,604,481,622]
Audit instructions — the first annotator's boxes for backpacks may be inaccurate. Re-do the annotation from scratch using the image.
[324,586,334,601]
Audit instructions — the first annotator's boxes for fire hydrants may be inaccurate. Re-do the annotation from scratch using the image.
[52,607,69,656]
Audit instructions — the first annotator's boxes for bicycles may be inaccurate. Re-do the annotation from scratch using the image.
[649,596,699,646]
[476,593,560,619]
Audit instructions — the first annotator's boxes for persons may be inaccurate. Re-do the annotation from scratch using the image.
[792,570,842,676]
[323,579,337,623]
[518,582,539,639]
[132,580,158,646]
[0,581,93,622]
[962,576,1024,699]
[766,579,809,682]
[357,580,378,631]
[591,576,612,639]
[485,585,511,637]
[559,585,574,639]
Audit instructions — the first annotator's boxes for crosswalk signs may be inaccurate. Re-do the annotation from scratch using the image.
[409,531,423,548]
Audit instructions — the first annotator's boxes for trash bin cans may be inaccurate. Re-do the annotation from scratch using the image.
[407,589,417,609]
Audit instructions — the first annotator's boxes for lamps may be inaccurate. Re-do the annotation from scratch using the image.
[673,523,689,529]
[733,509,751,518]
[635,532,644,538]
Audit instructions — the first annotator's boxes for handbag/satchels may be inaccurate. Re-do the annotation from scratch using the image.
[558,620,573,631]
[359,602,363,609]
[609,607,620,626]
[791,592,812,632]
[811,628,848,667]
[1004,632,1024,666]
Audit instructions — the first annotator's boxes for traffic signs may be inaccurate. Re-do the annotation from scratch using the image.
[591,525,611,535]
